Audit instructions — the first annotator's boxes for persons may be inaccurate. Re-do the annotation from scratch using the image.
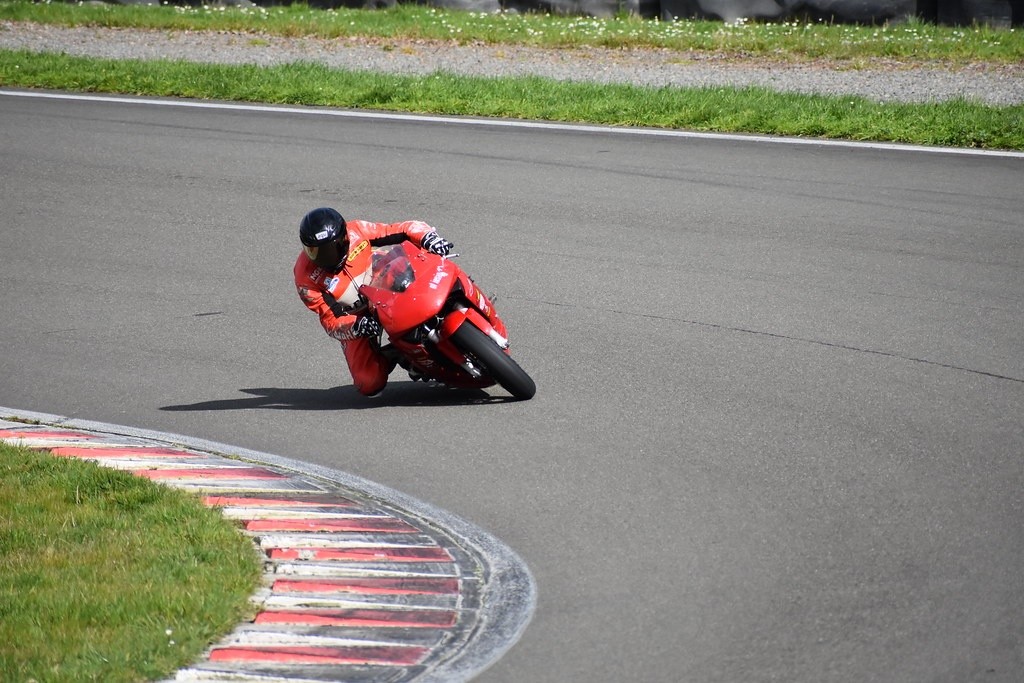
[293,208,454,396]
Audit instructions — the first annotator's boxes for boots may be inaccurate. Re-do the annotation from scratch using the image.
[385,356,410,374]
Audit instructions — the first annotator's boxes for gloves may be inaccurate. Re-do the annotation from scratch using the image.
[422,234,453,255]
[354,318,380,338]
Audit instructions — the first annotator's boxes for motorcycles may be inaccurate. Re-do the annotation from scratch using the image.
[333,238,537,401]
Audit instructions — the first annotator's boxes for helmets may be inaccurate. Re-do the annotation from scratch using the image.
[300,208,349,272]
